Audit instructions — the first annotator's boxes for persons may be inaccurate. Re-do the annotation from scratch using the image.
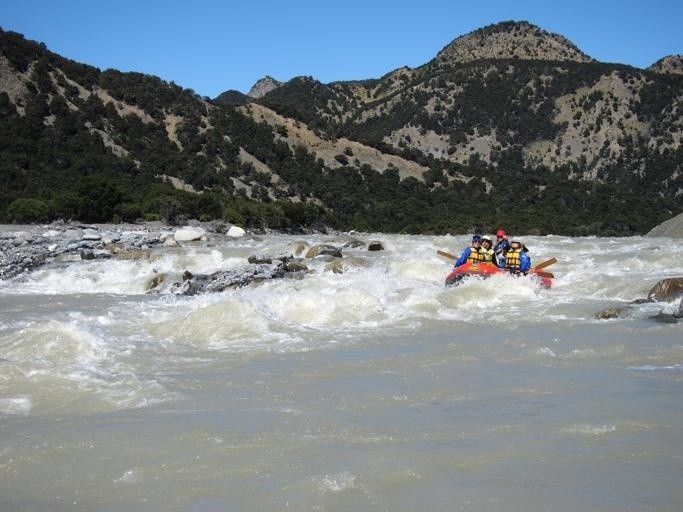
[454,230,531,277]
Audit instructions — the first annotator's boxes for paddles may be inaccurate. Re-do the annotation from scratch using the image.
[438,251,557,278]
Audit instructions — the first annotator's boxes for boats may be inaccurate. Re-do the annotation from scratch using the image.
[444,262,552,289]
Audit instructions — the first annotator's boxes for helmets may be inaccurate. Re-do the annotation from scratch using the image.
[482,235,492,241]
[511,236,522,247]
[473,236,481,242]
[497,230,506,237]
